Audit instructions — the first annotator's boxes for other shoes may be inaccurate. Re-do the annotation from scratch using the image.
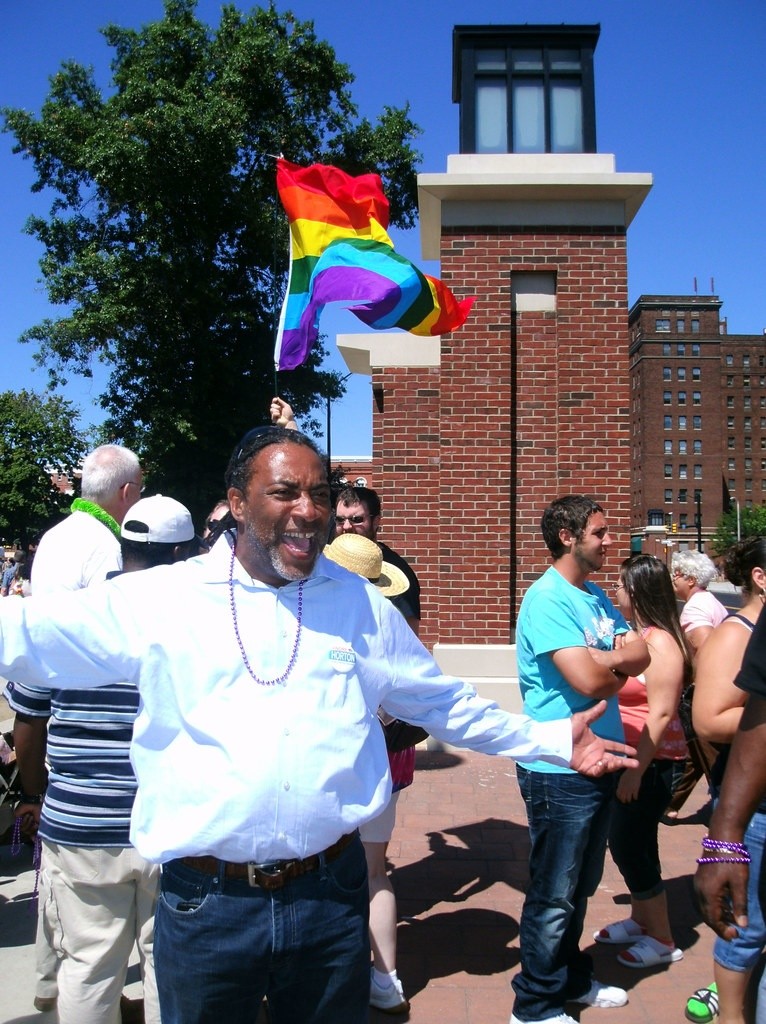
[660,806,678,825]
[34,996,56,1011]
[121,996,141,1024]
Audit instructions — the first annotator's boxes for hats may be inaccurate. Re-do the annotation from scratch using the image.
[120,495,195,543]
[322,533,409,598]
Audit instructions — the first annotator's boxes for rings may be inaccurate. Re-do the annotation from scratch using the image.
[597,761,604,766]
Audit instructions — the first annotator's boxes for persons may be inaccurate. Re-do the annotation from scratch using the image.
[694,601,766,1024]
[1,397,723,1024]
[691,536,766,1024]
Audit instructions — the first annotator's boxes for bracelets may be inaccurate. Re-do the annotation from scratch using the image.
[696,838,751,864]
[20,793,44,804]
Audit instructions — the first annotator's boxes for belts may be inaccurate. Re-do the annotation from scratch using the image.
[178,827,359,889]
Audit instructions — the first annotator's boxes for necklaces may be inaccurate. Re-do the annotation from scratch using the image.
[228,534,305,686]
[12,816,40,911]
[70,497,122,545]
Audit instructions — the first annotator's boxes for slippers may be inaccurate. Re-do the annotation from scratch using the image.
[617,939,683,967]
[593,920,647,943]
[685,987,719,1023]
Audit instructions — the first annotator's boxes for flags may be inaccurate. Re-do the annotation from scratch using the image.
[274,162,479,374]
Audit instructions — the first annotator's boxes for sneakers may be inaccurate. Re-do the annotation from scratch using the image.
[565,980,628,1008]
[369,967,407,1012]
[509,1012,579,1024]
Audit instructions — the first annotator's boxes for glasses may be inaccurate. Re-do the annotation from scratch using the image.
[333,515,374,526]
[670,573,684,581]
[120,480,146,493]
[612,583,625,591]
